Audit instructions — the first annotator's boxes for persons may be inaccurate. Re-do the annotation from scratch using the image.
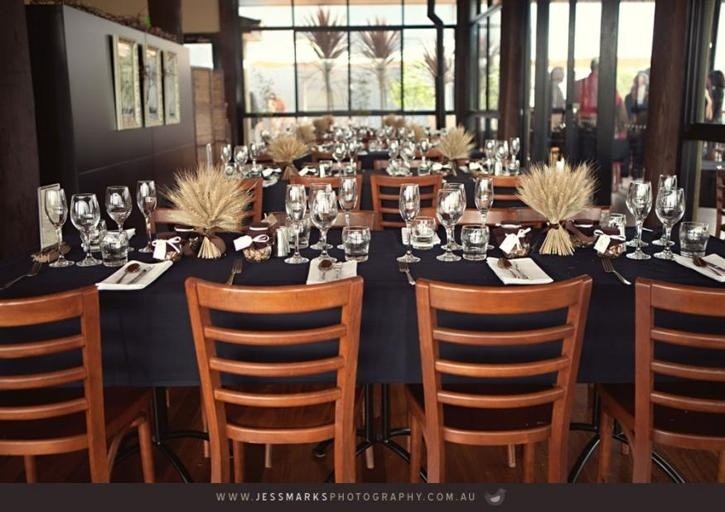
[574,55,627,193]
[624,70,652,177]
[548,65,567,111]
[705,67,725,159]
[254,90,297,143]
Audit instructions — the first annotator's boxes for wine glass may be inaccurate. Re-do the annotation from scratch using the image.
[45,190,74,267]
[485,140,496,176]
[509,137,520,175]
[71,193,102,266]
[396,184,421,262]
[476,177,494,249]
[311,192,338,261]
[333,143,346,176]
[436,189,464,261]
[652,175,678,246]
[347,143,358,168]
[441,183,467,250]
[334,125,408,141]
[496,139,508,176]
[137,180,158,253]
[337,178,360,250]
[250,143,260,170]
[625,182,652,259]
[420,139,430,171]
[389,140,398,176]
[105,186,134,252]
[221,144,231,173]
[284,184,309,263]
[235,146,248,164]
[653,188,685,259]
[308,183,334,250]
[401,141,413,175]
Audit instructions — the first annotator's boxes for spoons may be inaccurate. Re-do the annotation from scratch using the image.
[319,259,332,282]
[117,263,140,283]
[695,258,721,276]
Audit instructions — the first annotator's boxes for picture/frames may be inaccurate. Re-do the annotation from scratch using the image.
[112,31,144,132]
[140,42,166,128]
[163,49,182,126]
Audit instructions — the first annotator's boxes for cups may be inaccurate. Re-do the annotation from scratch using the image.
[103,232,129,267]
[608,213,626,249]
[285,215,310,249]
[343,226,370,262]
[680,222,709,257]
[319,160,332,177]
[461,225,489,260]
[412,216,435,249]
[81,220,106,252]
[624,236,649,247]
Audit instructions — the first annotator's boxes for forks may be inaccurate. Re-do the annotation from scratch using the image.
[0,262,43,293]
[498,258,519,278]
[602,256,631,284]
[226,263,242,286]
[400,264,415,285]
[687,254,725,272]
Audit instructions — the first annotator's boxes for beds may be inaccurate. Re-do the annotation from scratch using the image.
[8,223,725,487]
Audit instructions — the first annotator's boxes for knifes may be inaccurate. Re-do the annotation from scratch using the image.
[513,263,529,280]
[129,266,154,284]
[334,261,343,282]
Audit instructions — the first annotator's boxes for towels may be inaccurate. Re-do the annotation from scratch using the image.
[98,259,174,290]
[673,252,725,285]
[83,227,135,251]
[487,256,553,286]
[306,258,358,285]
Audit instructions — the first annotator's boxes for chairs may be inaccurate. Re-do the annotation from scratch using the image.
[596,278,725,485]
[182,275,365,486]
[368,175,443,227]
[478,175,537,208]
[0,287,158,485]
[404,273,595,484]
[287,174,364,215]
[223,177,263,225]
